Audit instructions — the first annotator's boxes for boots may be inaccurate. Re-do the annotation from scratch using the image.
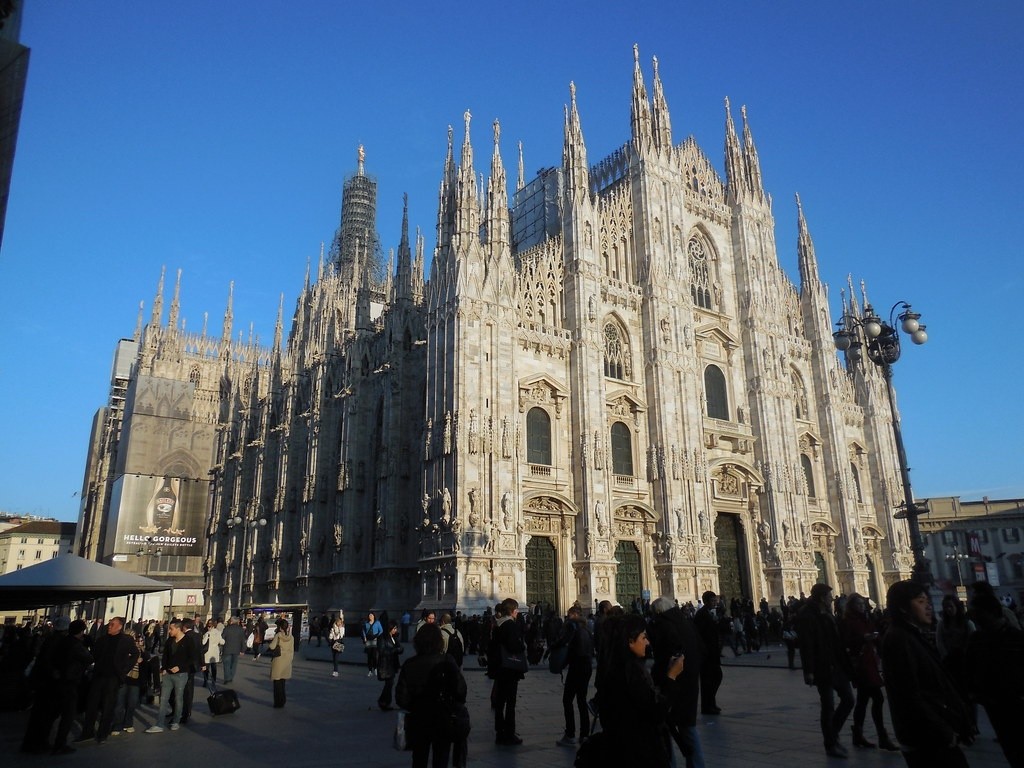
[877,728,899,750]
[850,724,877,749]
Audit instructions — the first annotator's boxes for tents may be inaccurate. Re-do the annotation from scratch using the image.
[0,553,174,639]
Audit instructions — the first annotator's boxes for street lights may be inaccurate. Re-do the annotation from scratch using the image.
[831,299,932,588]
[226,501,267,609]
[944,542,968,613]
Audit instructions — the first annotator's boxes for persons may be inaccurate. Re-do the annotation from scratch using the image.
[662,317,692,340]
[376,510,383,528]
[699,510,709,530]
[206,557,211,573]
[898,528,904,547]
[854,525,861,544]
[421,489,513,556]
[269,618,293,709]
[0,613,269,757]
[247,545,251,563]
[764,346,791,368]
[225,551,230,565]
[588,293,598,314]
[300,531,306,549]
[675,508,686,531]
[308,613,345,676]
[595,500,606,524]
[334,524,342,545]
[761,520,808,540]
[830,366,836,382]
[361,579,1024,768]
[271,537,276,555]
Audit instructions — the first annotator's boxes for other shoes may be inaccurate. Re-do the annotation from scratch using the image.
[494,730,523,744]
[224,679,233,684]
[273,701,285,708]
[94,736,105,744]
[831,729,847,747]
[366,670,372,676]
[110,730,121,736]
[71,733,96,744]
[374,669,377,676]
[170,724,179,730]
[700,703,721,715]
[824,747,847,757]
[51,744,75,755]
[377,697,394,712]
[331,670,339,677]
[144,725,163,733]
[122,725,135,734]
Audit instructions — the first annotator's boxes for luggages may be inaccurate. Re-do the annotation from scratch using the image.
[206,690,241,716]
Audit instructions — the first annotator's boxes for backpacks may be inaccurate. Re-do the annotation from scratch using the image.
[441,626,464,668]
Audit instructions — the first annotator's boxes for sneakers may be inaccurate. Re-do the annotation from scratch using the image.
[556,734,576,747]
[577,735,588,743]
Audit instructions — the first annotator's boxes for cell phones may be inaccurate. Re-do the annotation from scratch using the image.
[674,649,686,660]
[872,632,879,637]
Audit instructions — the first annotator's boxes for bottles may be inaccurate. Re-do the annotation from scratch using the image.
[968,620,976,632]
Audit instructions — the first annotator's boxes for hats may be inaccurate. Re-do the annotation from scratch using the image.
[68,619,86,634]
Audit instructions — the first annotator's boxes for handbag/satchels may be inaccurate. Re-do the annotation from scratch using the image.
[572,731,613,768]
[548,620,577,673]
[498,620,529,673]
[392,710,413,752]
[332,642,346,652]
[202,633,210,655]
[270,633,282,657]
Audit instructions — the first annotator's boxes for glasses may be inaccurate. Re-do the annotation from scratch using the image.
[139,639,143,642]
